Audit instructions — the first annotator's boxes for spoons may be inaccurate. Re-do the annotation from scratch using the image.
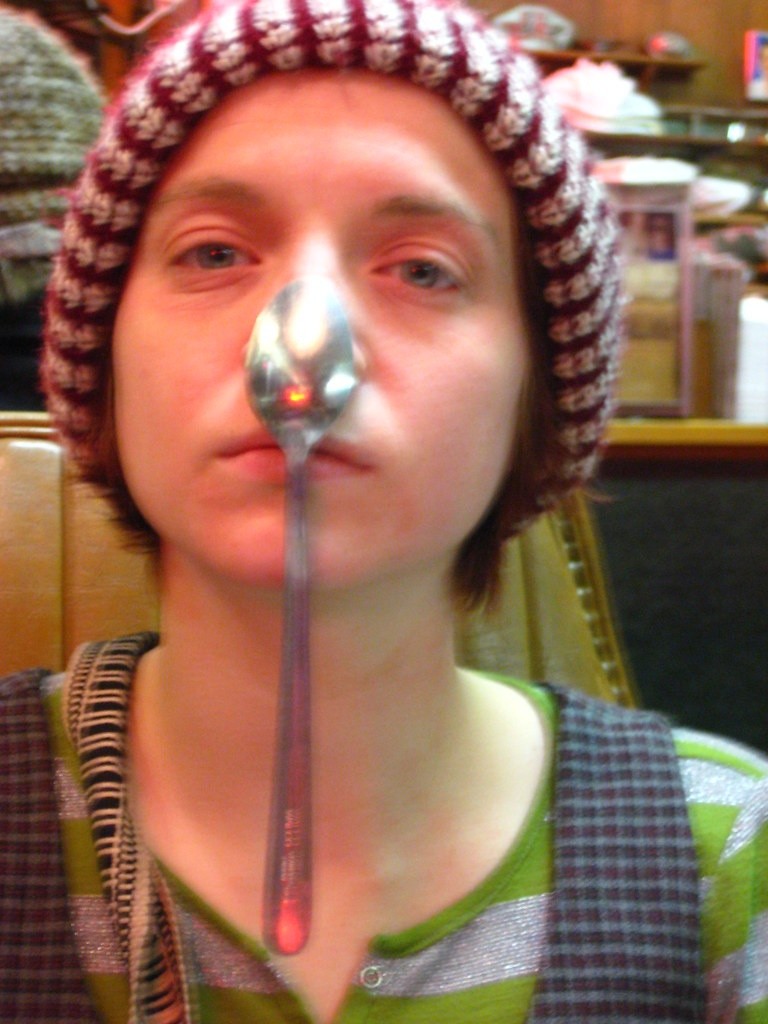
[245,273,361,958]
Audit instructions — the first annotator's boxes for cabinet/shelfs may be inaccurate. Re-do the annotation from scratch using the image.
[528,50,767,224]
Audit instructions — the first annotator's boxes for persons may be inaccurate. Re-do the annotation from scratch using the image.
[1,0,768,1023]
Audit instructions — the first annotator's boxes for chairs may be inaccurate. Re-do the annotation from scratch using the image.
[0,409,637,706]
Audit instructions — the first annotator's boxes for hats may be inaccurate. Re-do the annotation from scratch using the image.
[41,0,629,540]
